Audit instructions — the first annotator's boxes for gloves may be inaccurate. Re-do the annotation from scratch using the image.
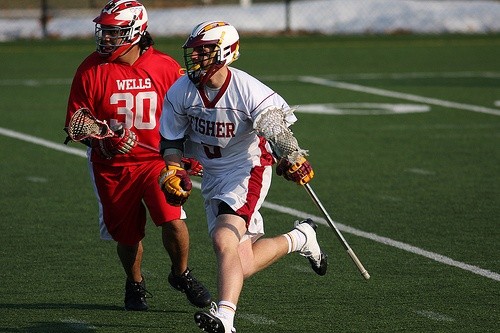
[102,122,138,155]
[181,158,204,176]
[158,166,192,206]
[276,149,315,185]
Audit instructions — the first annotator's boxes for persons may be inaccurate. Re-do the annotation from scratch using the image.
[66,0,212,311]
[157,21,328,333]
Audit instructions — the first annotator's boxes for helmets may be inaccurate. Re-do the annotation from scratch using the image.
[181,19,240,88]
[94,0,147,62]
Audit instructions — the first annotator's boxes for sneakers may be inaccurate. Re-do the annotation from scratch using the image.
[294,218,328,276]
[124,275,153,313]
[167,266,212,308]
[194,301,236,333]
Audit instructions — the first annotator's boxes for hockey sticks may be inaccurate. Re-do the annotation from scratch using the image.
[64,105,160,154]
[247,104,370,278]
